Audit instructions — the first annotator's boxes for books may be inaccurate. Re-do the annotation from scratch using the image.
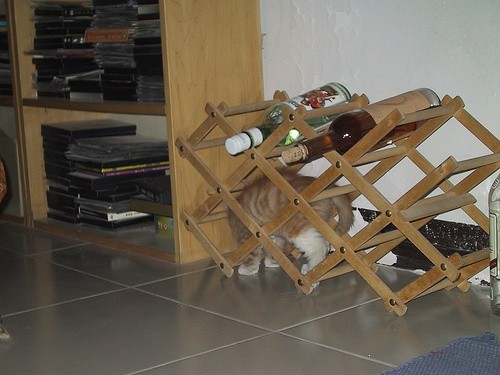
[0,0,166,102]
[41,119,173,231]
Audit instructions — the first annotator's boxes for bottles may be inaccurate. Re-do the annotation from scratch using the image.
[487,174,500,317]
[224,82,351,157]
[281,87,439,166]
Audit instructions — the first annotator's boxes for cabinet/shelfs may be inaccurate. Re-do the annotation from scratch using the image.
[11,0,265,264]
[175,90,500,315]
[0,1,28,226]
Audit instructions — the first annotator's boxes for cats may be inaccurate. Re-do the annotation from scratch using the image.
[227,167,355,296]
